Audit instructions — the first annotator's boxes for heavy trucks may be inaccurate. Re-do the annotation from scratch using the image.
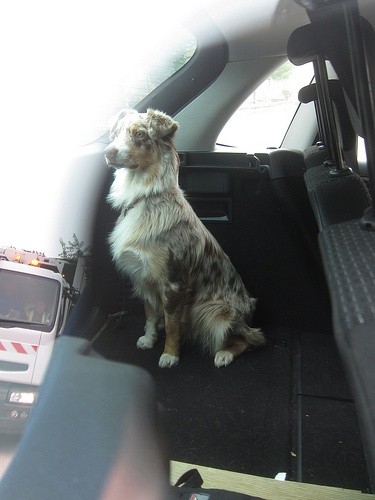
[0,243,71,443]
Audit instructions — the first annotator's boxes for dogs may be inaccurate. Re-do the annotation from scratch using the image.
[102,109,269,369]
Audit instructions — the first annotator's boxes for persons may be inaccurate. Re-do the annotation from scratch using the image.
[25,296,53,324]
